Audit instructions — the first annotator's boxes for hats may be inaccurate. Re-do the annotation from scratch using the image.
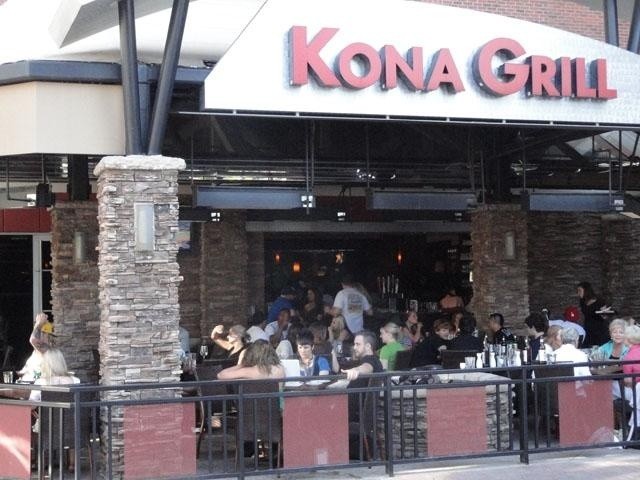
[35,322,57,338]
[562,305,580,322]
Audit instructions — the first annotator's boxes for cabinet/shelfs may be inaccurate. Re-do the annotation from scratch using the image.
[445,236,472,294]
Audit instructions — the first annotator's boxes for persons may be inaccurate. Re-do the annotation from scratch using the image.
[176,260,640,429]
[15,313,68,381]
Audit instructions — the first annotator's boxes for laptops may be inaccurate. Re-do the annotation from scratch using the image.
[280,359,304,387]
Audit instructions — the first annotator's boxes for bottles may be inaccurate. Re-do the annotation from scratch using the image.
[589,345,602,362]
[458,334,557,369]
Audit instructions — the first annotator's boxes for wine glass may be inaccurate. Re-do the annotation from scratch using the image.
[200,345,208,361]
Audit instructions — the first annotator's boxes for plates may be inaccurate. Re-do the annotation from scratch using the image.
[286,379,330,388]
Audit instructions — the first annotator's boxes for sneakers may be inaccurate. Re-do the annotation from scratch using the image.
[32,418,40,434]
[212,406,239,419]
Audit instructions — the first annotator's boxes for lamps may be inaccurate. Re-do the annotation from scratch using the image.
[133,201,156,252]
[300,120,317,216]
[210,209,221,224]
[502,229,516,262]
[72,230,86,265]
[336,189,346,221]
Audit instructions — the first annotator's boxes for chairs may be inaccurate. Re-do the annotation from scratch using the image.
[91,348,101,369]
[32,383,96,479]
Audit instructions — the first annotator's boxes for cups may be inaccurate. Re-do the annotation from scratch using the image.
[2,370,14,384]
